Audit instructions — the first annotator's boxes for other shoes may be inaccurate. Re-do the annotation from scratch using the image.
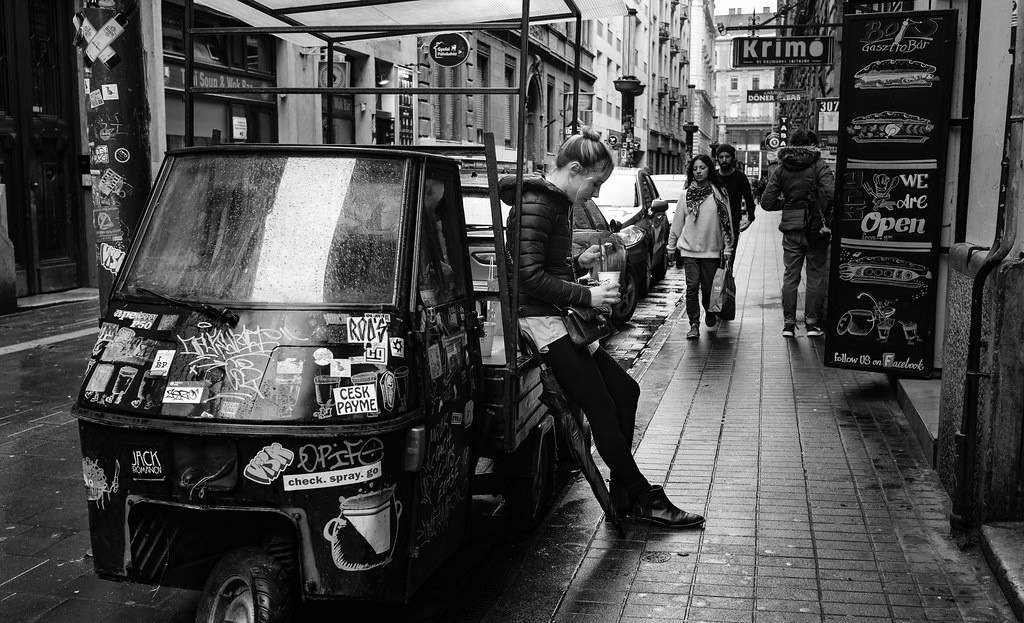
[686,324,700,338]
[807,324,824,337]
[705,313,716,327]
[782,324,799,336]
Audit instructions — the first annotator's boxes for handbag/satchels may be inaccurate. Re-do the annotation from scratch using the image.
[778,208,807,233]
[708,260,736,321]
[560,304,611,347]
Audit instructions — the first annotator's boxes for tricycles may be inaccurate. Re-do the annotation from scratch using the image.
[70,131,591,622]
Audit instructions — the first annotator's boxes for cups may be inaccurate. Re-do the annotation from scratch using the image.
[479,322,496,357]
[597,272,620,299]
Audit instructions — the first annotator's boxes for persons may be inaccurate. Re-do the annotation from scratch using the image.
[757,177,766,205]
[715,144,755,271]
[499,126,706,529]
[761,130,836,337]
[666,155,735,338]
[752,178,758,198]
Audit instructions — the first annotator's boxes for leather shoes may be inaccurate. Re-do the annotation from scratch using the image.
[605,480,632,522]
[632,485,705,527]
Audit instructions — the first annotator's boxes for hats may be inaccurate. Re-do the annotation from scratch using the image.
[717,144,735,158]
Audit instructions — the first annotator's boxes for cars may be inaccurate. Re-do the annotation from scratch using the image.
[590,167,672,326]
[459,167,640,298]
[649,174,688,226]
